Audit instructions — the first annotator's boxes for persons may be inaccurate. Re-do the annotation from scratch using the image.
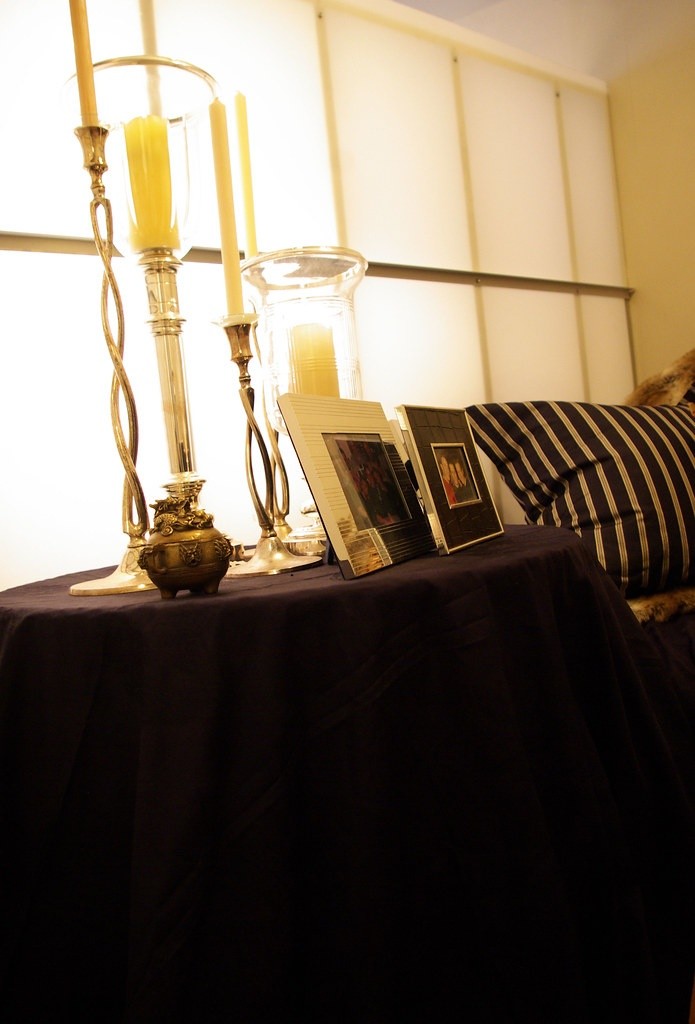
[440,454,474,505]
[339,443,407,524]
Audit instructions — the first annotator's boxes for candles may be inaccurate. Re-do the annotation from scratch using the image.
[124,114,182,252]
[70,0,98,127]
[232,90,257,259]
[211,97,257,324]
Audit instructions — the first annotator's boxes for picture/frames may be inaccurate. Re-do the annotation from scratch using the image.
[277,392,436,580]
[397,404,505,555]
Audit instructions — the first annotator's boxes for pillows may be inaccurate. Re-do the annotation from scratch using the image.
[465,401,695,599]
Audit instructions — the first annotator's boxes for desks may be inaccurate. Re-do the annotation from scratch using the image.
[0,525,695,1024]
